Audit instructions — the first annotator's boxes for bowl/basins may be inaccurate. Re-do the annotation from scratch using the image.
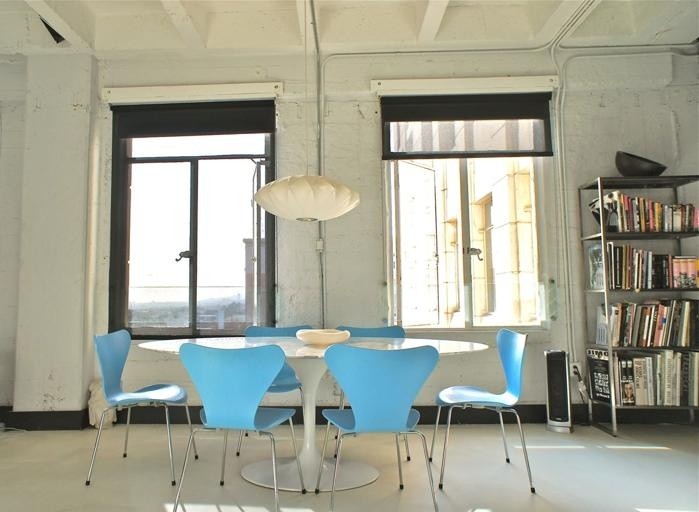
[615,149,667,177]
[295,327,351,346]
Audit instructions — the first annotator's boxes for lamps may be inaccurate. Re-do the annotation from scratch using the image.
[251,0,366,225]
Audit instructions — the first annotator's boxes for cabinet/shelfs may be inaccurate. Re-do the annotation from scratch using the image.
[576,172,699,438]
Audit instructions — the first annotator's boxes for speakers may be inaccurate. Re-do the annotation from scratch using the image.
[544,349,573,435]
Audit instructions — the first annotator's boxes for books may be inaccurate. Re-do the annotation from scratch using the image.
[585,190,699,407]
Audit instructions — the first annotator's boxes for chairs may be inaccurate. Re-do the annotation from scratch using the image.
[428,327,536,490]
[84,328,199,488]
[333,325,410,458]
[235,324,312,458]
[171,341,307,512]
[313,342,441,511]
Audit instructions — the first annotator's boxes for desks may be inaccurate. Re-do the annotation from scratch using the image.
[134,335,491,494]
